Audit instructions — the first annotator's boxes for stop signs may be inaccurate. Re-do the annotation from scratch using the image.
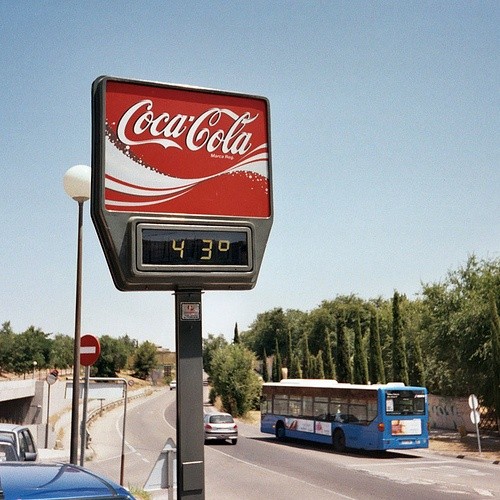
[75,335,98,368]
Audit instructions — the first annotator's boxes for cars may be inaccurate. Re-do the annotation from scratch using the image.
[0,426,36,461]
[201,412,238,446]
[0,462,137,500]
[169,380,177,390]
[0,436,19,461]
[33,361,37,379]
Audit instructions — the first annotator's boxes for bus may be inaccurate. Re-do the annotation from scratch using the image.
[257,376,431,453]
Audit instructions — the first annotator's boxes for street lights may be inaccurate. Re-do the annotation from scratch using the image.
[62,165,94,466]
